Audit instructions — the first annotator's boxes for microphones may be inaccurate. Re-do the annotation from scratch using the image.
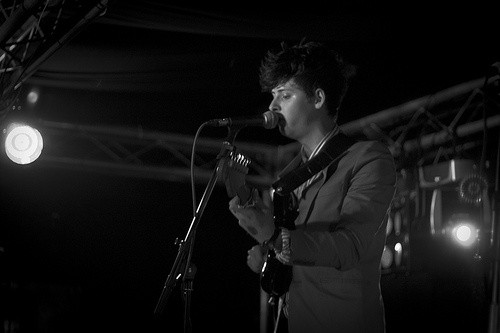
[207,111,278,130]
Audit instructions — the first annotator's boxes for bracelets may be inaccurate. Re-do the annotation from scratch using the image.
[273,227,292,265]
[263,224,282,250]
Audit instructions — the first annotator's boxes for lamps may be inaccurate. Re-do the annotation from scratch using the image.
[0,118,43,164]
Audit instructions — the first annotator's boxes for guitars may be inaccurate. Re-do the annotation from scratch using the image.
[217,152,300,297]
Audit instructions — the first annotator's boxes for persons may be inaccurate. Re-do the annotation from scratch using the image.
[229,43,396,333]
